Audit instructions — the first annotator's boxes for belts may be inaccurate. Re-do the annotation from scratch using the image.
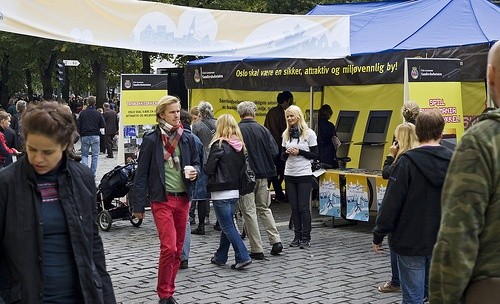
[168,192,185,197]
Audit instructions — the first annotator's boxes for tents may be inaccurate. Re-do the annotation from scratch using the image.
[186,0,500,228]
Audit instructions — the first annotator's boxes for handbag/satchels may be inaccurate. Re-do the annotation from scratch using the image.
[242,159,256,195]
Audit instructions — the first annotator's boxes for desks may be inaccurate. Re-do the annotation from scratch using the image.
[319,169,389,228]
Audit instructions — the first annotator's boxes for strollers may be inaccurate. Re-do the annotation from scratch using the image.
[96,159,143,232]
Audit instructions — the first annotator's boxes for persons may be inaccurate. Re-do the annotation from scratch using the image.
[0,40,500,304]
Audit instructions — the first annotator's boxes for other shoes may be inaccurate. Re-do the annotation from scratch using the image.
[209,255,227,266]
[158,296,178,304]
[188,216,196,225]
[204,217,210,225]
[106,155,114,158]
[190,226,206,235]
[269,242,284,255]
[250,251,265,261]
[180,260,189,269]
[289,238,303,247]
[299,240,311,249]
[231,258,252,269]
[377,281,400,293]
[213,222,221,231]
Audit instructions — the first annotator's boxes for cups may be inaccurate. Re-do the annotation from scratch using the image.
[184,166,194,178]
